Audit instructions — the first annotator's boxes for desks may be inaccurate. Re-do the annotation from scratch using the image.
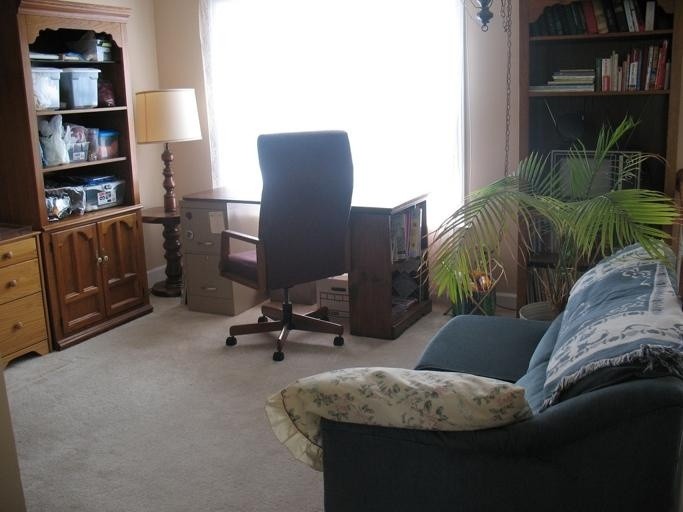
[180,185,433,340]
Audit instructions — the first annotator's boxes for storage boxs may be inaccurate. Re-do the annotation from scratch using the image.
[314,273,349,318]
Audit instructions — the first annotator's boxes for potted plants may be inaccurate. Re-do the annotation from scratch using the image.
[415,116,683,321]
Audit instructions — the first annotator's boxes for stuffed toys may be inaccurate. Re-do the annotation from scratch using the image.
[39,114,71,166]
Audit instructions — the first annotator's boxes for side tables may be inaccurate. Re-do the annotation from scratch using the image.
[141,207,181,297]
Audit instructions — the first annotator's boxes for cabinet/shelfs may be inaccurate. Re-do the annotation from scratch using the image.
[0,225,52,369]
[516,0,683,317]
[0,0,154,350]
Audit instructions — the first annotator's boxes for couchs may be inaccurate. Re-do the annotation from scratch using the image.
[266,239,683,512]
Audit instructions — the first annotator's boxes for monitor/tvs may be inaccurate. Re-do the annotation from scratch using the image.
[551,149,640,212]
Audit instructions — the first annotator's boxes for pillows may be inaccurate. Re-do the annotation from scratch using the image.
[266,366,532,471]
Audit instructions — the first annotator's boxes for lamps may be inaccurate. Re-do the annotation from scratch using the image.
[134,88,203,212]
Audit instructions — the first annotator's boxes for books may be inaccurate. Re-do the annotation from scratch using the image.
[523,0,670,97]
[388,207,422,318]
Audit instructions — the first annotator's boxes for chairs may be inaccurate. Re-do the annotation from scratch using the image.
[219,131,354,361]
